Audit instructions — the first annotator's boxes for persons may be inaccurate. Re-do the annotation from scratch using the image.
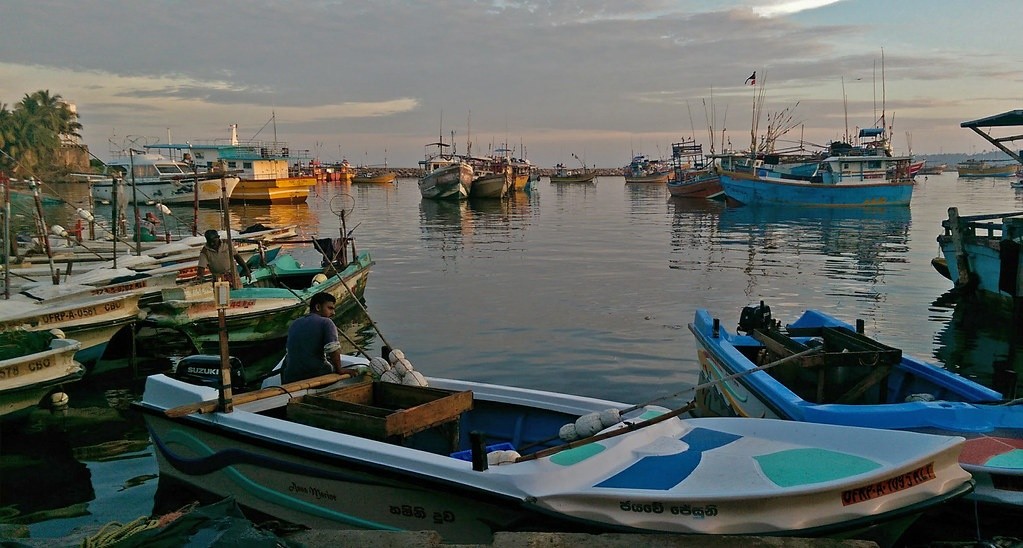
[194,230,252,289]
[133,213,164,241]
[281,292,360,385]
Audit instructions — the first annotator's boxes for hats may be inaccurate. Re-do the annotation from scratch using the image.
[205,230,217,239]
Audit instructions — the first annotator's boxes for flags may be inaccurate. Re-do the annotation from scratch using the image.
[745,73,755,87]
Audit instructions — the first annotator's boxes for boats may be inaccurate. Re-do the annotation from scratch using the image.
[688,302,1022,525]
[550,163,598,185]
[931,110,1022,320]
[622,44,1023,210]
[0,108,401,425]
[415,108,540,205]
[135,279,975,548]
